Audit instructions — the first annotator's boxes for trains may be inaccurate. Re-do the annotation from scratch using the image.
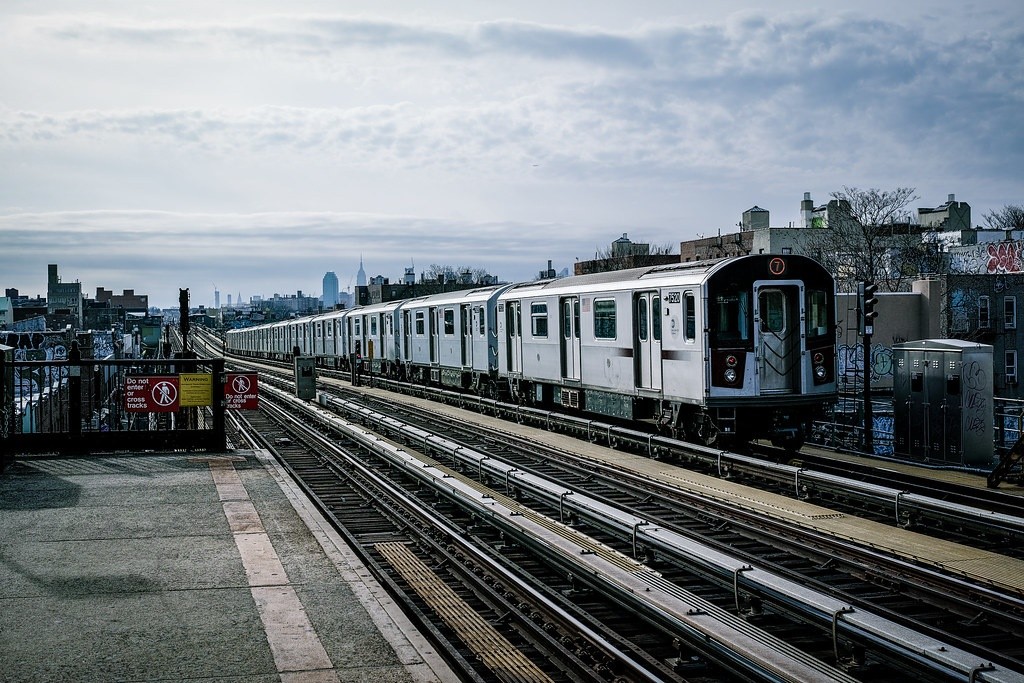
[224,254,841,446]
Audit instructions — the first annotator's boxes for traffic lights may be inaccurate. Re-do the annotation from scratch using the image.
[355,340,361,359]
[858,281,879,336]
